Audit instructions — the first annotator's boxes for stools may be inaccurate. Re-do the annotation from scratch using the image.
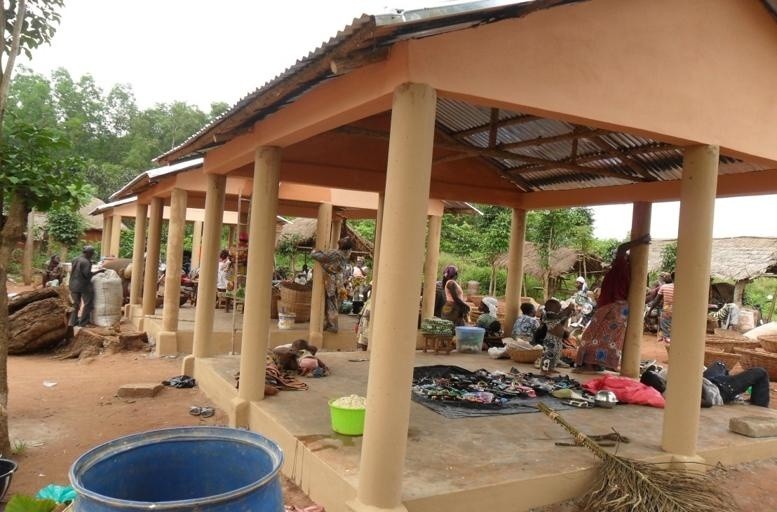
[421,333,453,355]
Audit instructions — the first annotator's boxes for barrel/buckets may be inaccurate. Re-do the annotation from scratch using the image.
[278,312,296,330]
[0,458,19,504]
[738,309,755,330]
[66,427,285,512]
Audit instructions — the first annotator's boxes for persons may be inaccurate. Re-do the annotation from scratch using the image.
[66,243,232,330]
[41,255,66,287]
[272,343,317,369]
[356,280,373,351]
[434,234,769,408]
[287,256,369,314]
[274,339,325,375]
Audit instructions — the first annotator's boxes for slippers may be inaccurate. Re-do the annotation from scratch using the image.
[190,407,214,417]
[534,358,540,368]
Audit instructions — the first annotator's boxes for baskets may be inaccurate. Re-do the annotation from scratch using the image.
[507,347,542,363]
[272,282,312,323]
[665,319,777,380]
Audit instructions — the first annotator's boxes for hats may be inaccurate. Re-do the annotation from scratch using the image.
[83,246,98,254]
